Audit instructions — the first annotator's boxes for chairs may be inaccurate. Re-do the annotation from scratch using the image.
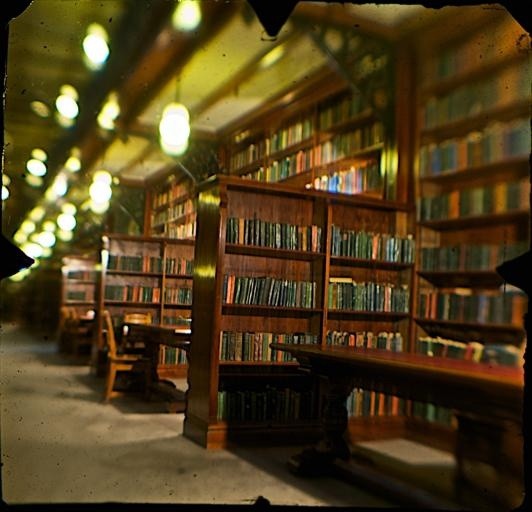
[122,311,151,350]
[101,310,139,403]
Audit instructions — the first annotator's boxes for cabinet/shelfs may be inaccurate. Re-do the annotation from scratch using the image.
[91,232,195,378]
[182,175,413,452]
[59,256,97,352]
[144,74,387,238]
[389,11,529,453]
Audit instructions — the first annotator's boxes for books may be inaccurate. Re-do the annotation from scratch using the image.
[217,212,416,420]
[68,270,86,300]
[416,12,532,370]
[106,255,196,365]
[150,182,195,239]
[229,90,386,196]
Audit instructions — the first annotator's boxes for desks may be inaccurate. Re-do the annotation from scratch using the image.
[123,321,191,401]
